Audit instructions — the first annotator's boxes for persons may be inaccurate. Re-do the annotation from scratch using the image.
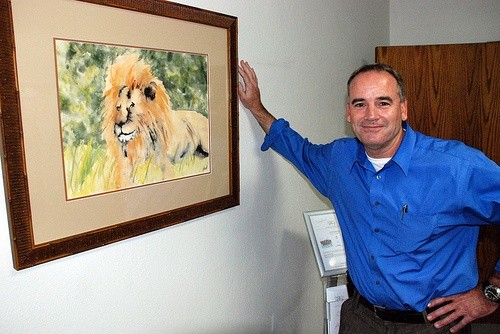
[236,60,500,334]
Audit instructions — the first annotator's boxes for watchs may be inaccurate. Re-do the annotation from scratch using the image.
[481,279,500,304]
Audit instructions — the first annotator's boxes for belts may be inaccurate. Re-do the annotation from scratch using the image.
[344,270,442,325]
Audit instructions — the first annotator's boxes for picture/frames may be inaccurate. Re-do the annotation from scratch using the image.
[1,0,242,272]
